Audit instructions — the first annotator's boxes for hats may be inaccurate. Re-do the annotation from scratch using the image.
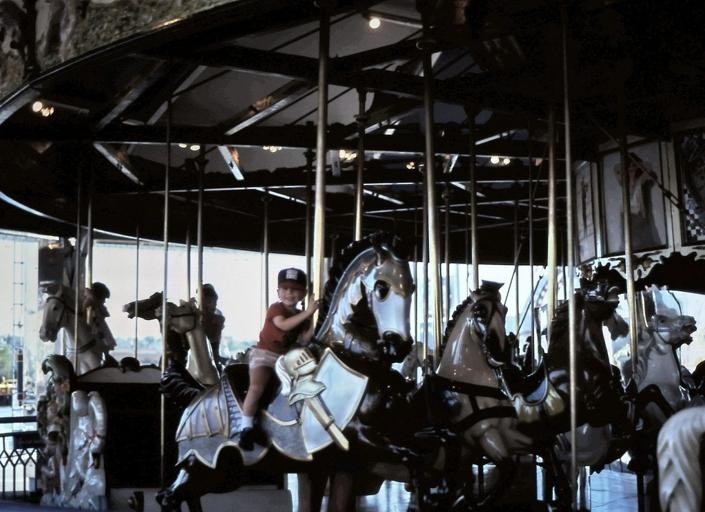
[278,268,307,291]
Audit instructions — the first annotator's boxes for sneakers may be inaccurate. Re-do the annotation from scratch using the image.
[239,427,254,451]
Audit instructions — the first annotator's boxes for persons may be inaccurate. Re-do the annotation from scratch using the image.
[179,284,225,378]
[238,268,324,451]
[84,282,118,366]
